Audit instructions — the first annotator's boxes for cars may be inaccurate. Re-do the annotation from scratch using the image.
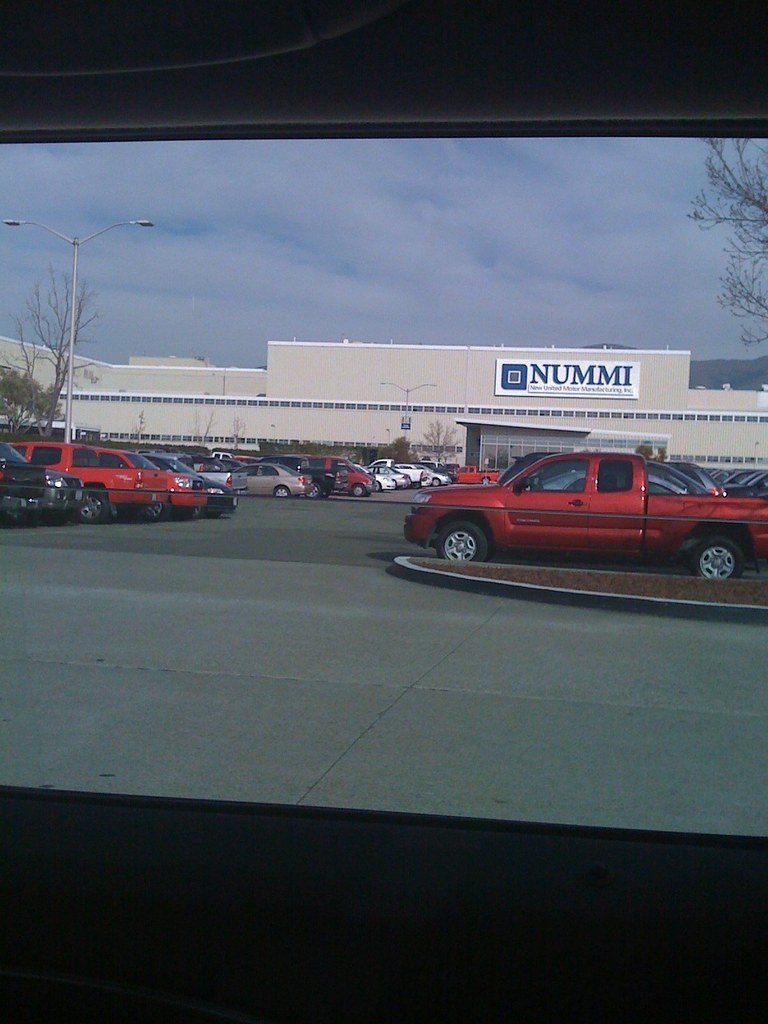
[0,439,768,580]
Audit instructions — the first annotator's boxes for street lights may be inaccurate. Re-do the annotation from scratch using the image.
[3,218,154,446]
[380,381,438,443]
